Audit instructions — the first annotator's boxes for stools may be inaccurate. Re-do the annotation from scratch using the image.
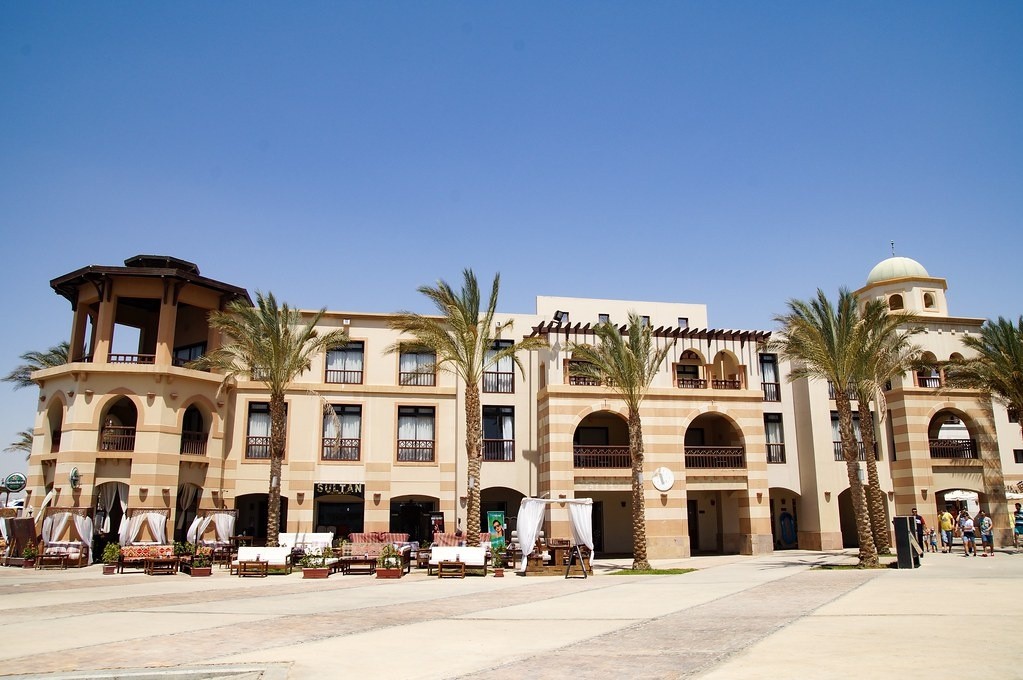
[219,545,236,568]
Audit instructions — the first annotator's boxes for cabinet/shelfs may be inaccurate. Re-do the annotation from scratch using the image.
[432,532,491,558]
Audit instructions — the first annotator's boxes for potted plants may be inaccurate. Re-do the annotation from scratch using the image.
[487,544,505,578]
[190,554,212,576]
[101,541,119,574]
[24,540,36,568]
[299,545,329,578]
[375,542,402,578]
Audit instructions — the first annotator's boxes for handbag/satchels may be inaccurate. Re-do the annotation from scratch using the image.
[964,530,975,536]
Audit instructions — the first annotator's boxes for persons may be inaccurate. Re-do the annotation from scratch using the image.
[431,523,441,534]
[977,510,994,557]
[1013,503,1023,546]
[493,520,504,538]
[911,507,976,557]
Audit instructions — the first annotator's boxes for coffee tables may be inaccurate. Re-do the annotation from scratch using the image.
[239,560,268,577]
[438,561,465,578]
[144,557,180,575]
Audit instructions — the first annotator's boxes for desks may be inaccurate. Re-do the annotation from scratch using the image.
[549,543,570,566]
[35,553,70,570]
[340,556,377,575]
[417,549,431,569]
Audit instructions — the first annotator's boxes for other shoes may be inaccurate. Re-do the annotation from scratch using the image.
[1013,549,1020,553]
[942,550,947,553]
[962,553,969,557]
[982,553,987,556]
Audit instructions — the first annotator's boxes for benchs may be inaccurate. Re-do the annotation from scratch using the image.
[348,533,409,549]
[428,546,487,576]
[41,541,90,568]
[1,554,25,567]
[528,550,543,566]
[306,547,339,573]
[493,547,521,569]
[202,540,233,558]
[277,532,336,557]
[571,544,591,565]
[338,543,411,576]
[230,547,293,575]
[117,545,176,573]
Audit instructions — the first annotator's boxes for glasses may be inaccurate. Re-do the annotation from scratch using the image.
[494,525,499,529]
[913,511,917,513]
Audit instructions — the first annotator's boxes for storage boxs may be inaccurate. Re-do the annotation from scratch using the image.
[894,516,923,569]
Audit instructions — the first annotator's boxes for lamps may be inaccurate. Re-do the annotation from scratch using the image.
[271,475,277,491]
[469,475,474,495]
[638,467,643,486]
[857,462,864,481]
[553,309,564,321]
